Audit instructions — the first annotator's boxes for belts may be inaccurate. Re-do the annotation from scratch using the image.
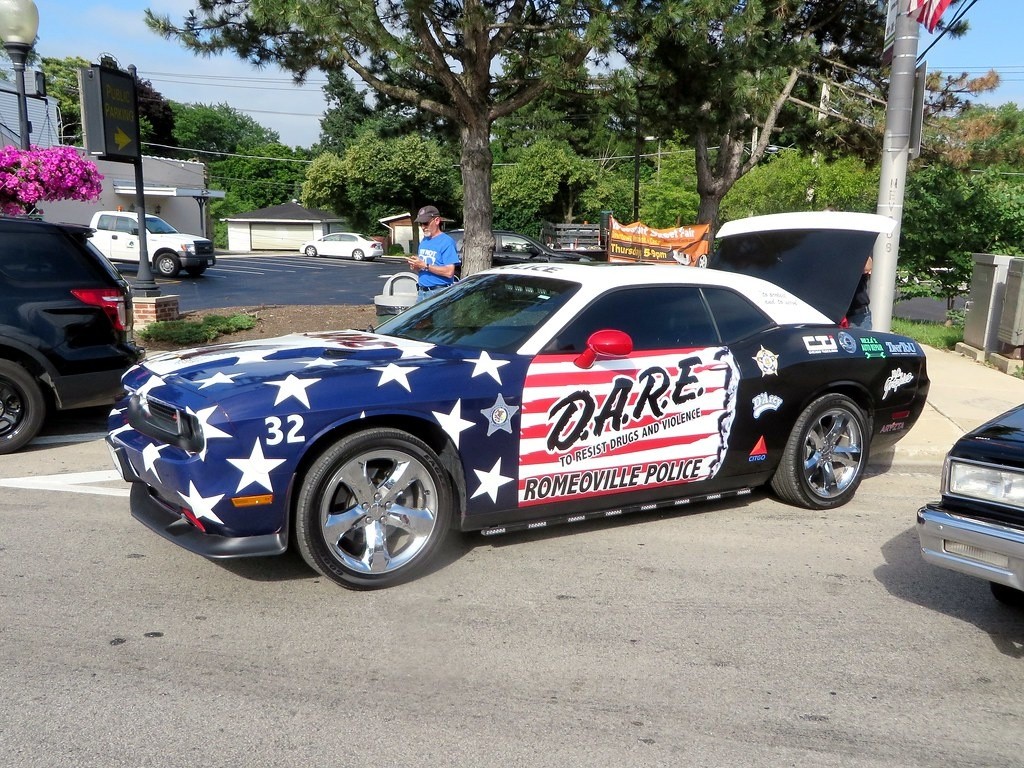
[847,305,869,315]
[418,285,448,291]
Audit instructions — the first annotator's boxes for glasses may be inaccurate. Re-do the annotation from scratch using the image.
[420,216,437,225]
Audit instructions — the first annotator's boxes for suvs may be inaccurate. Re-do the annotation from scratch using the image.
[87,205,216,279]
[444,228,595,281]
[0,214,147,455]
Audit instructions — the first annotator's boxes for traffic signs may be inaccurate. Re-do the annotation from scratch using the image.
[98,66,138,157]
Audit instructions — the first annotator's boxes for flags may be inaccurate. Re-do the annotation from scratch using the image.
[906,0,952,35]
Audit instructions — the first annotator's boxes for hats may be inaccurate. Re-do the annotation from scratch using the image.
[414,205,440,223]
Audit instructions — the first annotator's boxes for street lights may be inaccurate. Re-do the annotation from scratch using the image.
[0,1,39,214]
[644,135,661,187]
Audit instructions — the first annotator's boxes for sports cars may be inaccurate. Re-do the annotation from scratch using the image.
[105,211,932,590]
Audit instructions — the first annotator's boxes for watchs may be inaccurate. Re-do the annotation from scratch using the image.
[425,263,429,272]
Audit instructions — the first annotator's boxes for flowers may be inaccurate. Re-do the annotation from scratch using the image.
[0,145,105,218]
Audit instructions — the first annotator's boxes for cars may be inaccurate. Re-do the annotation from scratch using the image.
[300,232,384,260]
[915,404,1024,620]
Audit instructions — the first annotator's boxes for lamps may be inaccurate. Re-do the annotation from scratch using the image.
[129,203,135,212]
[156,204,162,214]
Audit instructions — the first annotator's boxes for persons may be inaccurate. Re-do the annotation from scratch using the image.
[405,206,459,305]
[843,254,873,332]
